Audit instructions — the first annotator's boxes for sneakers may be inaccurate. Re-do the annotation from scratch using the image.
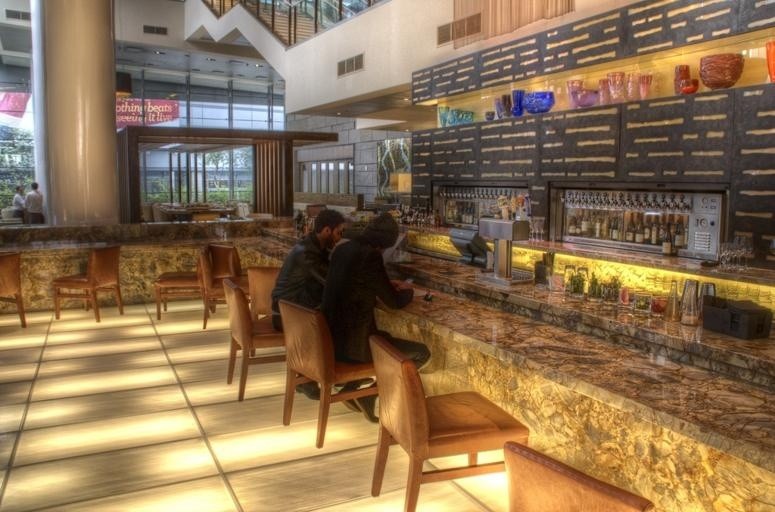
[293,377,320,401]
[335,378,379,424]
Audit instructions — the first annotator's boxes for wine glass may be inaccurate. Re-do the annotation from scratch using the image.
[713,234,755,274]
[527,216,545,242]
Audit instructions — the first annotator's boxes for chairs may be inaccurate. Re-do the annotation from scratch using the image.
[368,335,529,512]
[246,267,282,357]
[0,251,27,328]
[277,299,378,449]
[197,245,250,330]
[222,278,287,402]
[152,271,210,320]
[51,245,124,322]
[209,243,249,313]
[503,440,655,512]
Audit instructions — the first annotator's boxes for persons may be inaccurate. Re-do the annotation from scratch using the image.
[320,210,432,424]
[24,182,46,224]
[12,186,26,218]
[268,209,345,401]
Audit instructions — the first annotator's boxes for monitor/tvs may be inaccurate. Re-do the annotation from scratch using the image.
[306,204,327,218]
[449,228,493,267]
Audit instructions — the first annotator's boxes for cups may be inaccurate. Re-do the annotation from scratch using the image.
[445,200,475,225]
[764,39,775,85]
[510,89,525,117]
[673,63,692,97]
[485,111,494,122]
[437,106,475,130]
[495,93,511,120]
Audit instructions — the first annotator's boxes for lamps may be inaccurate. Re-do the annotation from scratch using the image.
[115,71,132,96]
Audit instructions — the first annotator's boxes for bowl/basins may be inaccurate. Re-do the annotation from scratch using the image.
[698,52,746,91]
[678,79,700,95]
[521,90,556,114]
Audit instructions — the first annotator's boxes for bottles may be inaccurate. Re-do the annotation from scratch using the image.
[533,260,719,327]
[564,207,689,257]
[565,69,654,111]
